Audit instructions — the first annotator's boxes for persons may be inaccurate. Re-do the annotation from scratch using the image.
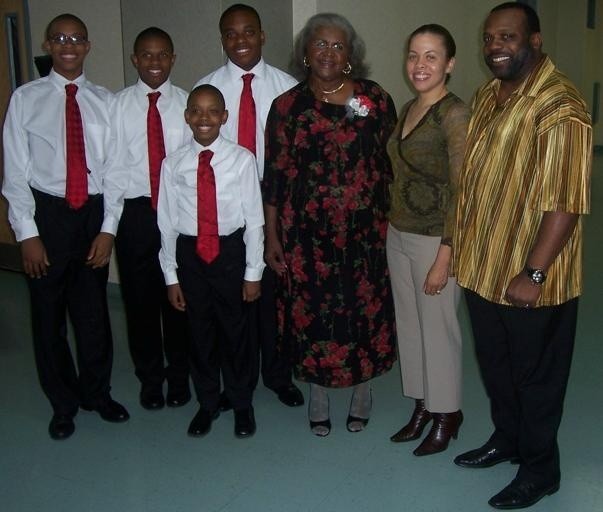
[449,3,594,510]
[387,25,473,456]
[115,28,191,411]
[263,12,398,436]
[157,84,266,438]
[3,15,130,441]
[191,5,305,412]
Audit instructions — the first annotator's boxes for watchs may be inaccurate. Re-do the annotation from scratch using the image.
[523,265,546,285]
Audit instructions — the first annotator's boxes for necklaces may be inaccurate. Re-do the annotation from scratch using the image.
[313,75,350,102]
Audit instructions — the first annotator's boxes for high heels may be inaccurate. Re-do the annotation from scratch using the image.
[390,398,434,442]
[412,409,464,457]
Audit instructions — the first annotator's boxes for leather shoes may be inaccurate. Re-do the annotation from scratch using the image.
[487,469,560,509]
[234,406,256,438]
[219,391,232,410]
[187,405,220,436]
[263,377,304,407]
[138,371,167,411]
[453,442,513,470]
[165,366,191,407]
[80,392,130,423]
[49,397,79,441]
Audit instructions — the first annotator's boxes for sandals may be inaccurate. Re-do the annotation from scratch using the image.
[309,391,331,436]
[347,389,372,432]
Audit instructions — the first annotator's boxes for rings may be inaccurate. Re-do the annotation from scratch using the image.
[436,290,441,293]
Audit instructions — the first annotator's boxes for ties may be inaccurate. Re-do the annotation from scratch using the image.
[238,73,257,157]
[196,149,220,264]
[65,83,89,211]
[146,91,168,211]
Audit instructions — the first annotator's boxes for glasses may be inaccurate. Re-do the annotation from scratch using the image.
[46,32,87,49]
[309,40,349,50]
